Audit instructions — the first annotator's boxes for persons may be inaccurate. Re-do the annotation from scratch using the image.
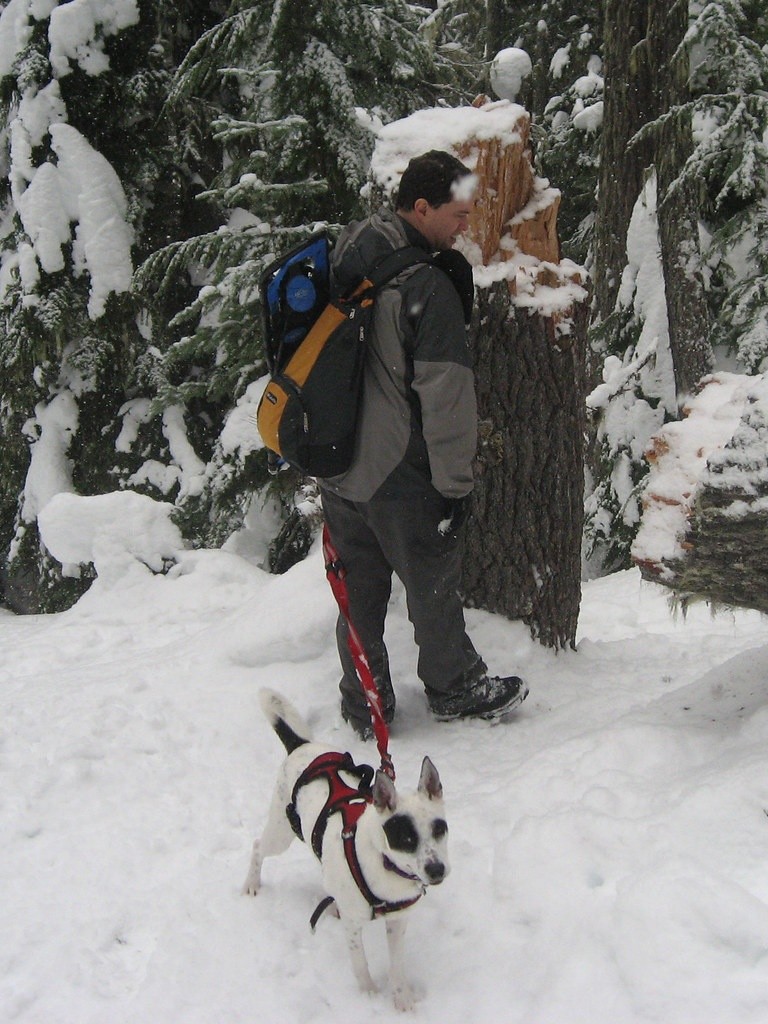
[319,150,528,742]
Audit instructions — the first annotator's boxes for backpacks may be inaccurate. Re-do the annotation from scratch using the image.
[253,229,340,373]
[255,246,438,479]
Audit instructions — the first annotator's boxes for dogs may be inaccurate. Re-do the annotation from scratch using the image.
[239,692,454,1016]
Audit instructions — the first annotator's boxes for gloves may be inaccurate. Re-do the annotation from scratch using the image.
[444,493,473,536]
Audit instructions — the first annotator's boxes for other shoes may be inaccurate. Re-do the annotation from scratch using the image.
[430,676,527,723]
